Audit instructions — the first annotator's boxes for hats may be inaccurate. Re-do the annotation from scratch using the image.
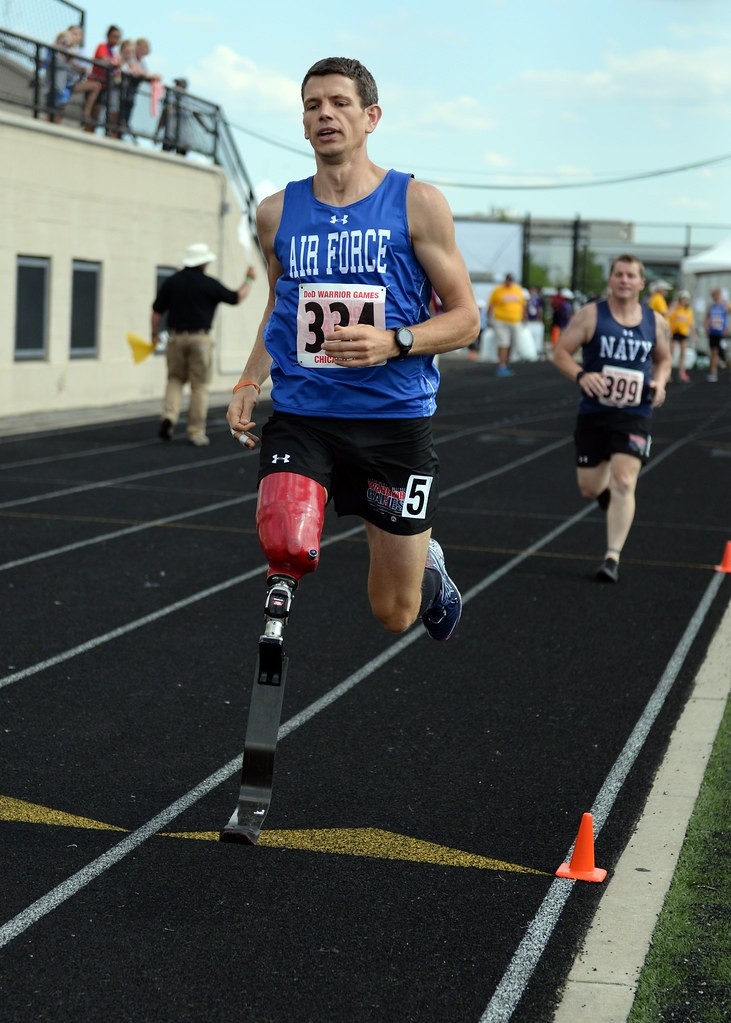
[182,243,216,267]
[560,288,574,300]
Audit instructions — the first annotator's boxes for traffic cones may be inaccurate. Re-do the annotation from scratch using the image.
[555,811,607,882]
[714,540,731,574]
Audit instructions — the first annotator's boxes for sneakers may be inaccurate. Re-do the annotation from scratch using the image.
[422,537,463,642]
[597,558,619,582]
[598,487,610,511]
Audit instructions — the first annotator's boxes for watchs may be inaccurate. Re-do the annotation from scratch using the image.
[390,326,414,360]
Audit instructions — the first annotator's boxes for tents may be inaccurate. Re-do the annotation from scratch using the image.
[682,238,731,340]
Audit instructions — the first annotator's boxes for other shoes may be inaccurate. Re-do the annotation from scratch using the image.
[192,436,210,447]
[680,373,689,383]
[497,368,512,378]
[707,374,717,382]
[159,418,175,443]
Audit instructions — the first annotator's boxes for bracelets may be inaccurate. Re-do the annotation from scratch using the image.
[233,381,261,395]
[246,277,252,284]
[576,370,586,384]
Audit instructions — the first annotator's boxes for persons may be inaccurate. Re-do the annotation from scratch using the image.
[152,244,257,445]
[644,277,731,382]
[551,254,673,581]
[469,274,606,377]
[226,57,482,846]
[46,25,205,156]
